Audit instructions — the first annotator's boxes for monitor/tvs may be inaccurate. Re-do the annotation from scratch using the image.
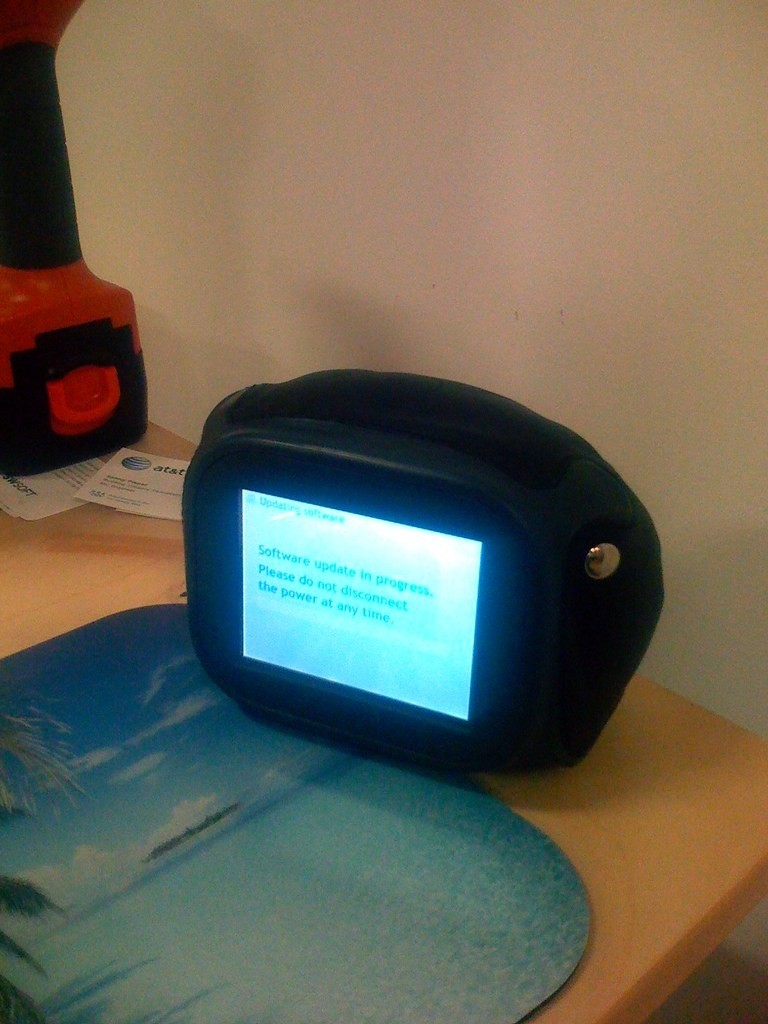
[194,430,527,747]
